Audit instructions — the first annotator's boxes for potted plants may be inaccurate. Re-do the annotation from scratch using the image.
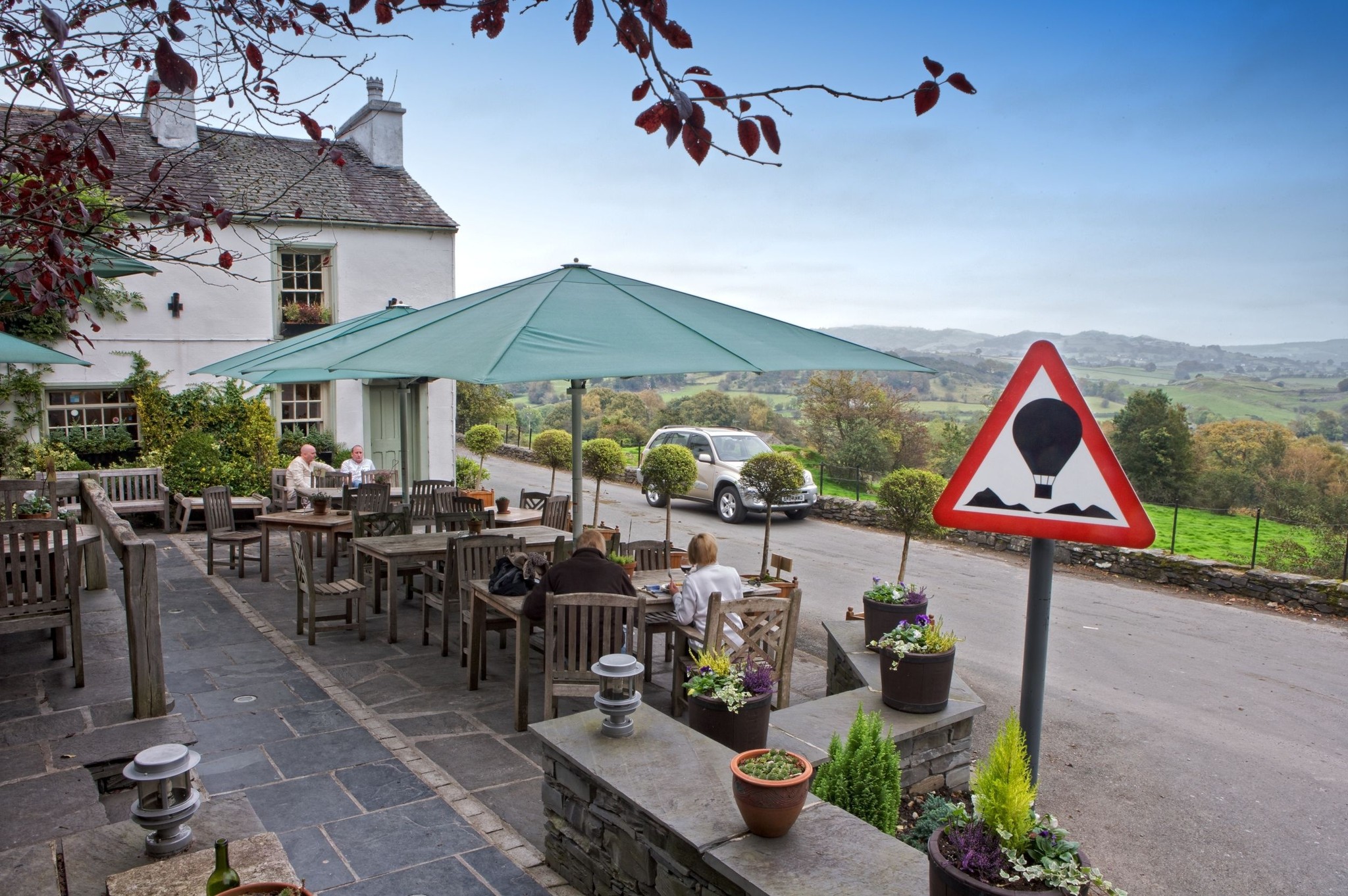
[370,472,391,494]
[495,496,511,514]
[608,550,636,583]
[466,507,484,535]
[18,495,52,540]
[310,493,331,516]
[730,748,813,839]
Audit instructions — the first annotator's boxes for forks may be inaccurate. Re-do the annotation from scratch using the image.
[668,568,672,579]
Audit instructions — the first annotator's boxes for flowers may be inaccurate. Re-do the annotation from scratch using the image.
[683,638,783,716]
[863,575,935,606]
[863,614,968,672]
[936,794,1079,888]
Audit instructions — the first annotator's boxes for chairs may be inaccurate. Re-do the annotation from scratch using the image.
[695,440,710,451]
[675,438,687,447]
[723,439,735,454]
[200,468,804,724]
[0,479,86,688]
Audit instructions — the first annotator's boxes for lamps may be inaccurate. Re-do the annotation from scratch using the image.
[591,653,645,739]
[123,743,202,857]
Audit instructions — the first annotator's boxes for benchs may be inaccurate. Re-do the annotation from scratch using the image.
[172,492,271,534]
[270,467,344,512]
[34,466,172,534]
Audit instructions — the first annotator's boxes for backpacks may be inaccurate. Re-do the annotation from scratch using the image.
[488,548,530,595]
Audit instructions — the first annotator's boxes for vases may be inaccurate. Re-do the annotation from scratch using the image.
[878,633,956,716]
[863,591,930,655]
[927,823,1090,896]
[686,676,773,754]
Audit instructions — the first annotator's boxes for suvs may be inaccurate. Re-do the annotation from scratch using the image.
[636,426,820,524]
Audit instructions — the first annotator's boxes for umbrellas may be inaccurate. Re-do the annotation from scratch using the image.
[188,258,940,541]
[0,330,95,369]
[0,218,162,281]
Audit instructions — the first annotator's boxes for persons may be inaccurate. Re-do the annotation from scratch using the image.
[669,532,745,662]
[285,444,337,503]
[523,529,639,669]
[340,444,377,488]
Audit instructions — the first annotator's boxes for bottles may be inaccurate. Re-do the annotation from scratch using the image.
[205,838,241,896]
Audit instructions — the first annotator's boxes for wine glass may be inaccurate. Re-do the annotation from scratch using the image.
[301,496,309,515]
[680,556,692,578]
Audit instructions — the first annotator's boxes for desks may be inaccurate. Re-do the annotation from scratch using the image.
[291,487,446,557]
[255,508,380,587]
[461,505,561,529]
[467,568,782,733]
[4,523,101,661]
[351,526,573,644]
